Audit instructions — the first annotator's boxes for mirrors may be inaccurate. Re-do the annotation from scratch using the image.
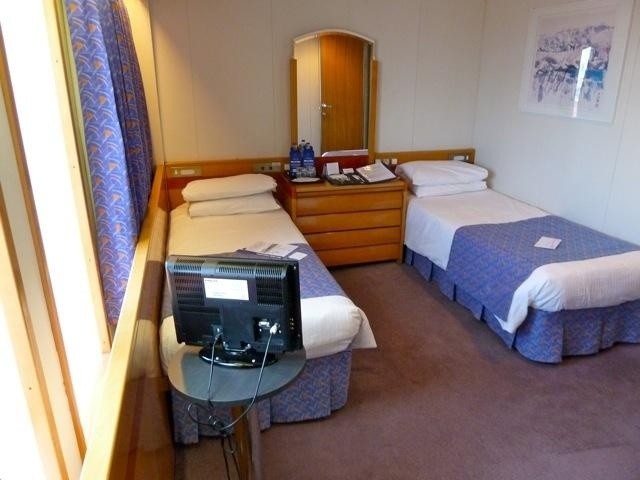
[290,28,379,159]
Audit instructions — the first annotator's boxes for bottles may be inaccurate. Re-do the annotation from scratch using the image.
[288,143,301,178]
[302,143,315,170]
[299,140,306,149]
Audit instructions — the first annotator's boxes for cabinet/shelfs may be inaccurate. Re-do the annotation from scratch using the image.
[281,171,409,271]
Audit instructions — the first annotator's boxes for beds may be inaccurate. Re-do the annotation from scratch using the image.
[375,149,640,362]
[160,159,356,444]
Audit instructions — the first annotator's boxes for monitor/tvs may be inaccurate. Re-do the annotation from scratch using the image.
[164,254,304,368]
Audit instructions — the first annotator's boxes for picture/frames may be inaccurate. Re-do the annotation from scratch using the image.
[517,0,633,124]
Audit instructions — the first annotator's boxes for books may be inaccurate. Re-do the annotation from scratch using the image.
[355,162,397,184]
[239,242,299,258]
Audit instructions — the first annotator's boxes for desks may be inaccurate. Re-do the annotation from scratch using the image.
[166,344,308,479]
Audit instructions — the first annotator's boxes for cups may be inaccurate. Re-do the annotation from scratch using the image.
[307,166,316,179]
[296,166,305,181]
